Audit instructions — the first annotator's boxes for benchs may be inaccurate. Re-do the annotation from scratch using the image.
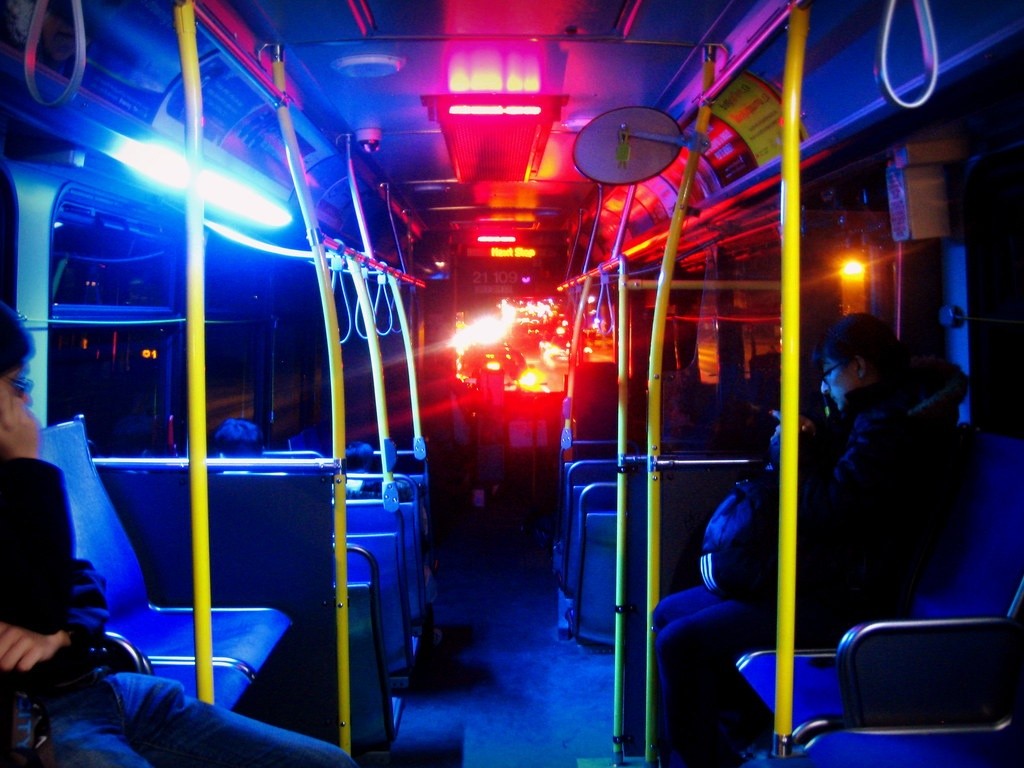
[548,431,1024,768]
[38,413,430,752]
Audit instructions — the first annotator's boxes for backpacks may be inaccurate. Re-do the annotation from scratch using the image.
[700,455,778,601]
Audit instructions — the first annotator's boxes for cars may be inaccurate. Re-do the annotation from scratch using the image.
[455,298,594,391]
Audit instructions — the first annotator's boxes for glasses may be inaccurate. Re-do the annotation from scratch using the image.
[0,373,32,394]
[821,357,853,384]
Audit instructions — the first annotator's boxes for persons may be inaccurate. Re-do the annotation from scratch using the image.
[652,313,967,768]
[214,418,264,452]
[333,440,441,652]
[0,300,361,768]
